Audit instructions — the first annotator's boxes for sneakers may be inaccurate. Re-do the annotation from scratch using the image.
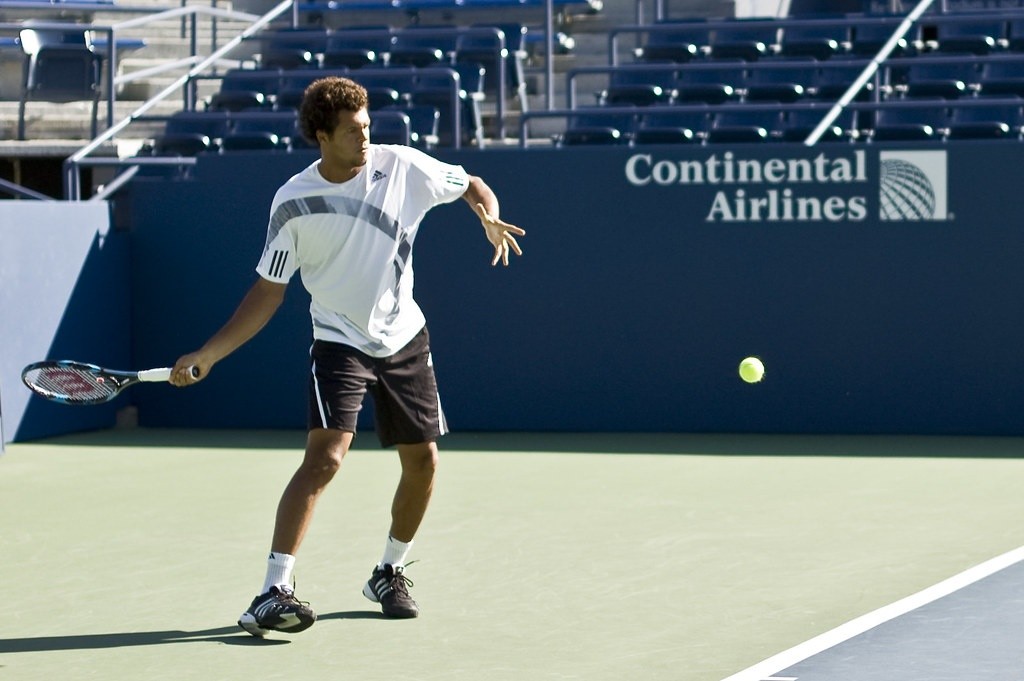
[363,562,419,617]
[238,575,317,636]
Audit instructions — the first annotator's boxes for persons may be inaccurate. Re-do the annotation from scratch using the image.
[168,77,526,637]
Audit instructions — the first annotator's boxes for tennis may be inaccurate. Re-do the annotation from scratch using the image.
[738,357,765,383]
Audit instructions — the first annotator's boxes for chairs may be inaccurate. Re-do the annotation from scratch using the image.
[131,0,1024,159]
[22,31,103,98]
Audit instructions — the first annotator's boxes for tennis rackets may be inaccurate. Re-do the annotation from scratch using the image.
[21,360,200,406]
